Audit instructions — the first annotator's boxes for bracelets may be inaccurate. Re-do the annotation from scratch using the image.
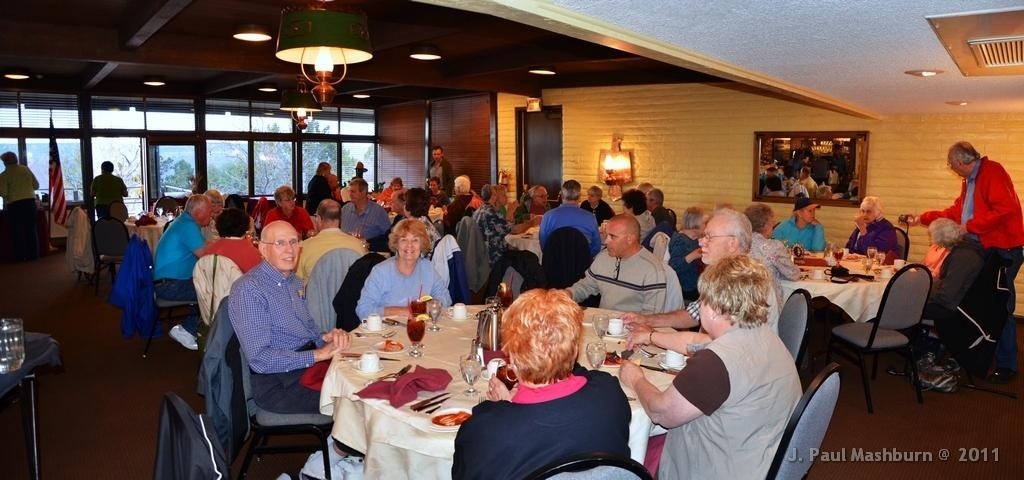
[649,329,657,345]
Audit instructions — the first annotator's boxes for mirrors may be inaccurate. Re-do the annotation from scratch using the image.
[597,146,634,187]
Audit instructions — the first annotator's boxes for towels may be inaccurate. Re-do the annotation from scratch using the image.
[356,365,453,410]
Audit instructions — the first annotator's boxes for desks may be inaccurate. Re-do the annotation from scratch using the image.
[760,250,928,389]
[0,316,64,480]
[320,295,713,479]
[0,203,59,263]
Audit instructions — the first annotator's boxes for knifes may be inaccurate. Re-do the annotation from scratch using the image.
[408,392,453,415]
[382,317,405,327]
[341,352,401,362]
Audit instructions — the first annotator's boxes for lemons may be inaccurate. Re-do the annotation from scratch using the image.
[415,313,431,322]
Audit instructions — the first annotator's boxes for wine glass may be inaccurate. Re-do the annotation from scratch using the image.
[403,297,483,397]
[156,207,164,219]
[824,240,886,275]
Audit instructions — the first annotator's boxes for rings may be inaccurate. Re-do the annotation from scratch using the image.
[343,332,347,336]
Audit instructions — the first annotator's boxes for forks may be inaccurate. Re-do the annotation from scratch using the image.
[364,363,412,391]
[354,329,401,339]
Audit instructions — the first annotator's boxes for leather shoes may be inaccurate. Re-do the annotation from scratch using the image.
[989,367,1018,385]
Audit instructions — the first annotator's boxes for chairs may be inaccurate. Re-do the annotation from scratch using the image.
[823,262,933,410]
[518,451,657,480]
[776,289,818,372]
[924,246,1017,400]
[762,358,837,479]
[190,296,355,480]
[154,382,242,479]
[884,224,911,261]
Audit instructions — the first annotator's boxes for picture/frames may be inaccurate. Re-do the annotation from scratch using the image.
[750,128,871,208]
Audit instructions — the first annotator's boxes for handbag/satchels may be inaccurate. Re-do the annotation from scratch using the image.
[912,350,962,394]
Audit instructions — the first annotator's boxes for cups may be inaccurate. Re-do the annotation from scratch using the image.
[486,358,506,379]
[167,213,173,221]
[356,352,380,371]
[585,313,687,372]
[495,364,517,392]
[812,269,823,279]
[0,316,27,376]
[880,260,907,277]
[360,314,382,332]
[485,285,513,309]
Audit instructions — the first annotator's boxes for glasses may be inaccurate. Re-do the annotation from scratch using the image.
[313,214,323,221]
[279,198,296,202]
[945,161,956,169]
[701,232,737,243]
[261,237,300,247]
[536,193,549,199]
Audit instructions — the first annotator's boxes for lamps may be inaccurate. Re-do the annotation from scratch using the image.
[615,153,630,185]
[3,64,30,84]
[528,62,554,80]
[142,76,166,87]
[603,149,617,193]
[230,0,442,135]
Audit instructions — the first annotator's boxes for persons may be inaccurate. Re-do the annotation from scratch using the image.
[616,208,782,358]
[450,288,633,479]
[897,141,1023,386]
[617,256,804,478]
[227,220,355,479]
[1,150,41,262]
[87,145,1024,315]
[355,218,452,322]
[153,193,213,352]
[885,217,990,377]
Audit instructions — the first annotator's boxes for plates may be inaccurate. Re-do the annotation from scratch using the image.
[425,409,473,433]
[368,341,409,355]
[358,324,386,333]
[350,361,384,373]
[480,370,489,382]
[808,274,828,280]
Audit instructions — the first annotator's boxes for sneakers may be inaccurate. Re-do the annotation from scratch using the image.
[168,324,199,352]
[298,453,347,480]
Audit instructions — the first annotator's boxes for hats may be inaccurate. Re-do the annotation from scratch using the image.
[794,198,822,216]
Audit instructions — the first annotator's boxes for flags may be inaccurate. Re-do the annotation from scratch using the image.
[48,115,69,231]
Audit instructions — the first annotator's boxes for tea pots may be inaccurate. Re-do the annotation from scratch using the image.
[475,306,502,351]
